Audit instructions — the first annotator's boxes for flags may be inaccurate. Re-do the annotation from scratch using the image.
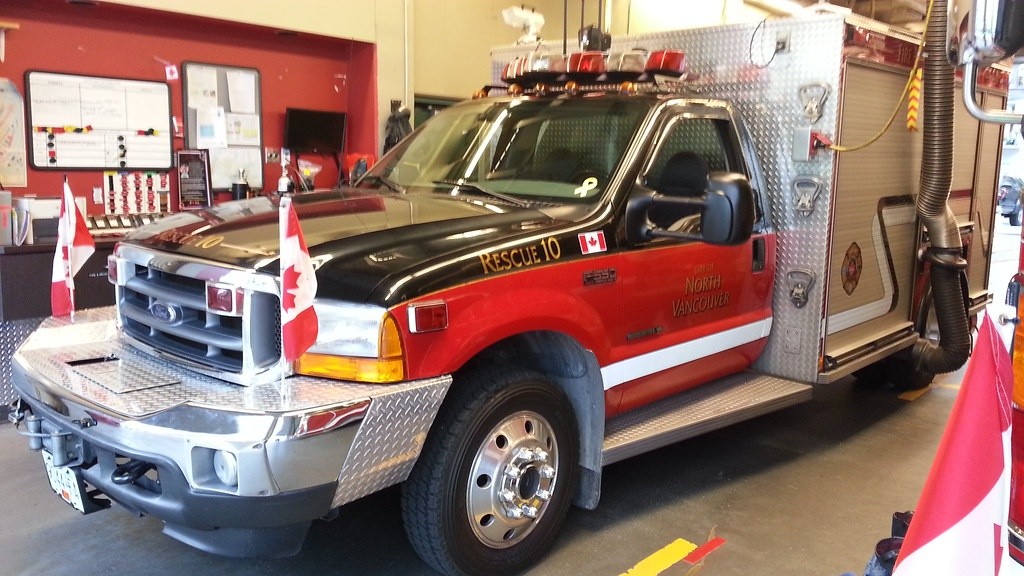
[279,196,317,362]
[891,310,1013,576]
[50,183,96,314]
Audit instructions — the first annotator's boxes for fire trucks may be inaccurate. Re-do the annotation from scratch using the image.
[12,15,1011,576]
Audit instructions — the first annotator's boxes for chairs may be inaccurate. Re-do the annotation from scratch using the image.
[537,147,582,183]
[648,151,709,230]
